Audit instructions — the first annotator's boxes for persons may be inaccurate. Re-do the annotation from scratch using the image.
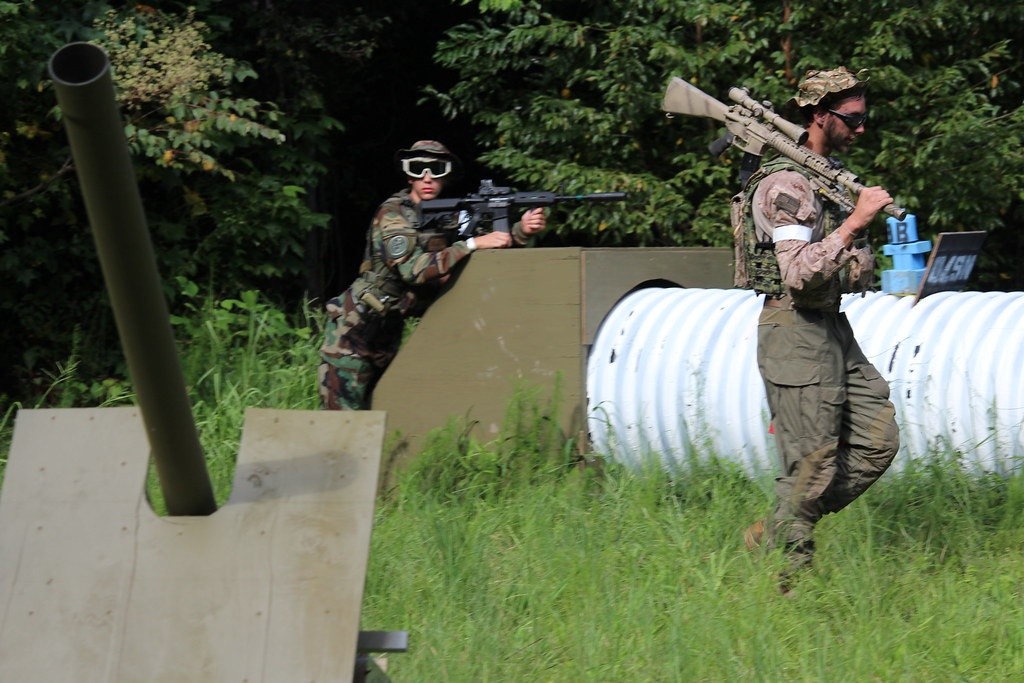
[726,65,899,604]
[317,139,547,411]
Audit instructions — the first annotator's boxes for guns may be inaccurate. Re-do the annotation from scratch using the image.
[661,77,908,222]
[417,178,632,235]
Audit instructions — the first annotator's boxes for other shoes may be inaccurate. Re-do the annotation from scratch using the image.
[745,519,791,595]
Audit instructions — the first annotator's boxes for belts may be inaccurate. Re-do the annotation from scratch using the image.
[763,299,806,311]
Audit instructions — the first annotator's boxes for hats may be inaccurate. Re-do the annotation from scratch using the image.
[783,66,871,117]
[398,140,451,155]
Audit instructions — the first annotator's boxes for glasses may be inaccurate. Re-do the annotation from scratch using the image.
[819,106,868,130]
[400,157,452,178]
